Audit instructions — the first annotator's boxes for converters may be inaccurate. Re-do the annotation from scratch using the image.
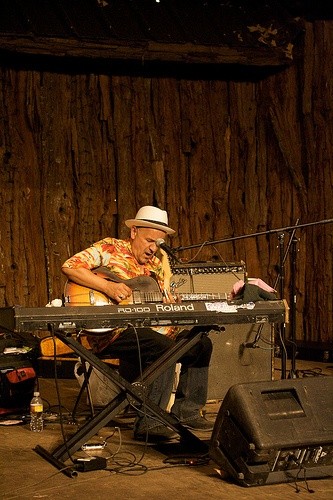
[73,455,107,472]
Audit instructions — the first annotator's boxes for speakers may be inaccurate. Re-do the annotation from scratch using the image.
[208,375,333,487]
[185,322,274,404]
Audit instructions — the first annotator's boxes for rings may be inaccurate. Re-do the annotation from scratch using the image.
[119,294,123,299]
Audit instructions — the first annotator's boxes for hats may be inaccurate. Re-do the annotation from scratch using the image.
[125,206,176,235]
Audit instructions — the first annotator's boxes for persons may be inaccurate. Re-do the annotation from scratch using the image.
[60,206,215,439]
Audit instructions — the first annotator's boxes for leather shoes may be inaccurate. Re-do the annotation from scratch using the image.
[181,416,215,431]
[134,425,180,439]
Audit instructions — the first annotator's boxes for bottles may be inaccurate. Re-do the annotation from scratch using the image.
[30,392,43,433]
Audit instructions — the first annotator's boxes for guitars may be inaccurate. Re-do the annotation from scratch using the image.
[64,266,233,353]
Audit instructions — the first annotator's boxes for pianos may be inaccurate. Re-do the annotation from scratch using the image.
[0,300,291,333]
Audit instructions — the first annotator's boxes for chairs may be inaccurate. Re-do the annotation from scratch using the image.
[38,333,120,435]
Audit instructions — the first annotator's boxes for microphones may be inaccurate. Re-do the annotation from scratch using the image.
[155,238,180,262]
